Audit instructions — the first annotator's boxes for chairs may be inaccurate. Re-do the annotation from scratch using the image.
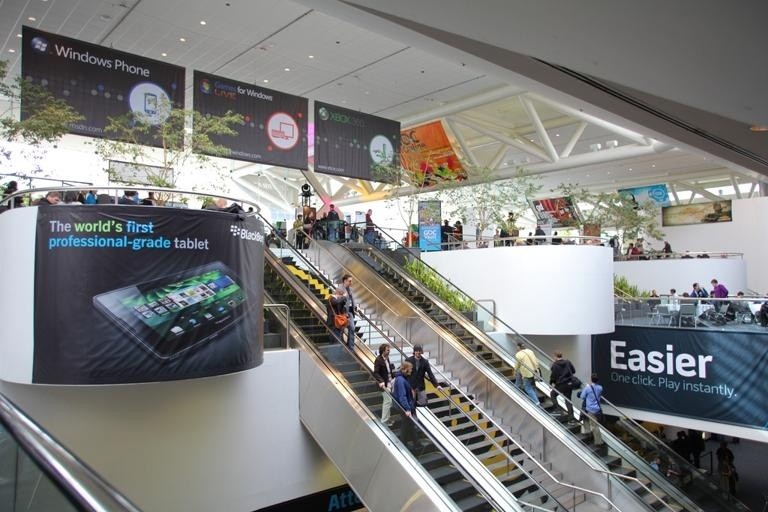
[614,299,763,328]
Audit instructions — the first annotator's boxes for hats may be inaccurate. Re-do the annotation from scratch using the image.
[517,342,526,349]
[414,344,423,354]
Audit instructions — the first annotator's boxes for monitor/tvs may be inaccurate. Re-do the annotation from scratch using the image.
[92,259,259,362]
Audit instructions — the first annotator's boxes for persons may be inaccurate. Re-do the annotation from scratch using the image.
[671,432,688,460]
[711,279,728,305]
[635,238,641,247]
[670,289,676,296]
[391,361,423,447]
[718,455,737,495]
[715,440,734,461]
[697,255,702,258]
[378,232,385,241]
[365,209,376,245]
[373,344,394,390]
[564,239,571,244]
[710,291,716,313]
[3,181,22,208]
[513,343,541,406]
[571,240,577,245]
[452,221,463,246]
[380,377,397,427]
[142,191,157,206]
[652,425,667,445]
[441,219,452,250]
[87,190,97,204]
[666,463,682,488]
[647,290,661,311]
[625,243,633,259]
[320,212,327,221]
[682,293,690,297]
[326,289,349,345]
[36,192,59,206]
[609,235,619,252]
[548,351,576,426]
[404,344,445,405]
[663,240,672,257]
[335,274,357,350]
[620,192,639,209]
[78,190,87,204]
[634,448,647,459]
[651,457,668,467]
[703,251,709,259]
[578,372,603,448]
[681,249,693,258]
[754,292,768,327]
[700,203,730,221]
[600,243,605,246]
[327,204,339,220]
[681,431,692,441]
[527,231,533,245]
[304,217,315,224]
[535,225,546,245]
[57,191,82,205]
[732,291,753,314]
[246,206,253,213]
[552,231,564,245]
[293,215,304,249]
[617,430,634,447]
[689,283,709,298]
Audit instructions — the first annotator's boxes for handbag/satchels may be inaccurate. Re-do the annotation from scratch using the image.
[416,391,428,406]
[567,364,582,389]
[581,416,592,435]
[329,299,350,329]
[590,386,605,423]
[524,351,541,381]
[734,470,740,484]
